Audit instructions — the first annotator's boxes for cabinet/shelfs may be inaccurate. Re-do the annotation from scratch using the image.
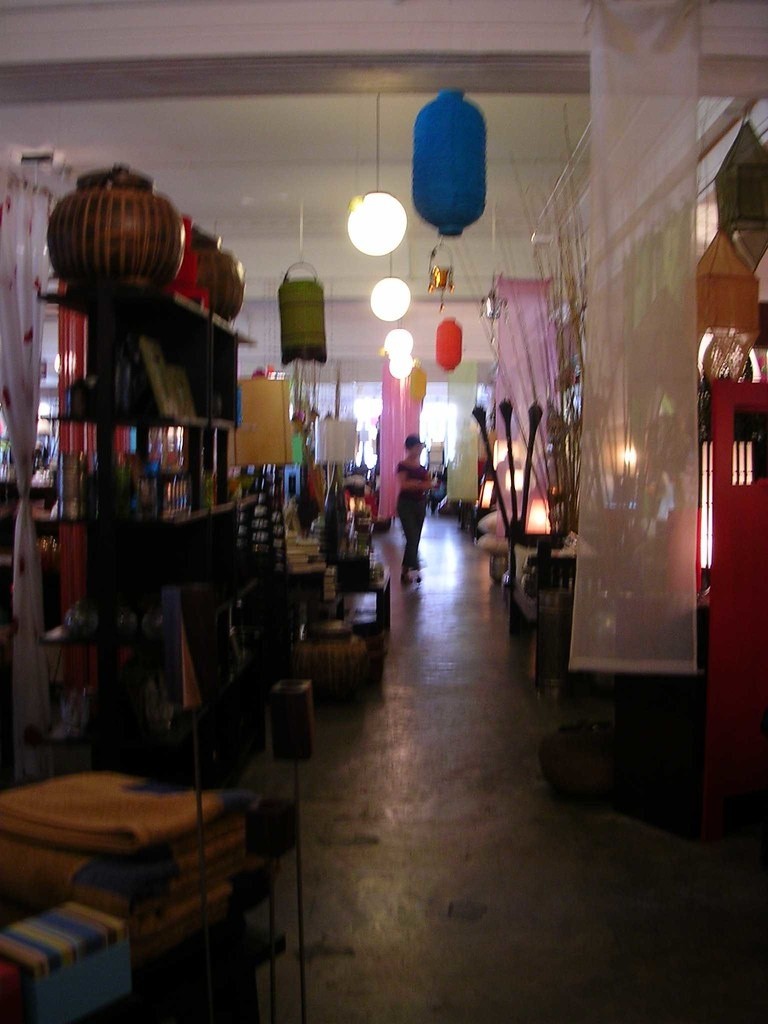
[39,280,265,789]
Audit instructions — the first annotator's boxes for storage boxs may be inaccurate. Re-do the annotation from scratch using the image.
[0,901,132,1023]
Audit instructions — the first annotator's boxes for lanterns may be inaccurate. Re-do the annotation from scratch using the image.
[412,87,487,236]
[436,318,461,372]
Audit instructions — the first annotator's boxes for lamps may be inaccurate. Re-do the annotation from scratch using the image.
[347,92,416,379]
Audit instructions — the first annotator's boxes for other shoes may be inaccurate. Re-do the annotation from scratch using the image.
[410,571,422,583]
[400,573,413,583]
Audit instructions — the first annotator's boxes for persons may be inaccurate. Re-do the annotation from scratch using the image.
[395,434,432,584]
